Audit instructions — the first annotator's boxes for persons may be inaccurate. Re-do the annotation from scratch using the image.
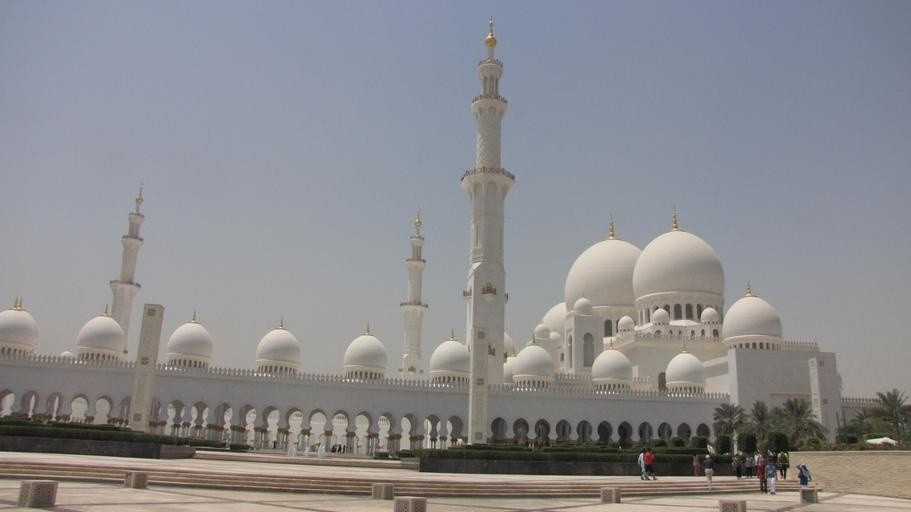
[693,451,702,477]
[704,454,715,491]
[797,462,814,484]
[641,447,659,480]
[638,447,651,480]
[732,446,790,494]
